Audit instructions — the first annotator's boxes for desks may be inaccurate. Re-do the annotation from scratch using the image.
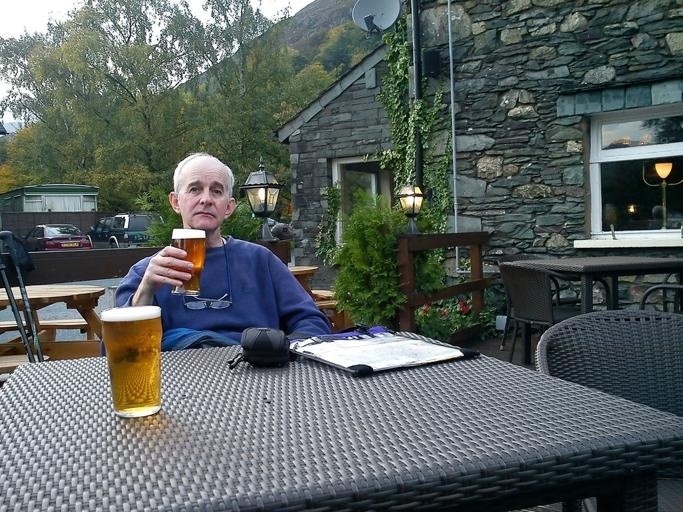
[0,285,104,360]
[288,266,319,302]
[0,332,682,511]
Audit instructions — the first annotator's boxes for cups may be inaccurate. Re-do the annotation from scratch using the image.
[170,228,206,297]
[101,304,162,418]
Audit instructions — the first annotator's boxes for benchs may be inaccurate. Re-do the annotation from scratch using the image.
[0,319,94,341]
[311,289,336,300]
[0,354,48,374]
[314,300,349,332]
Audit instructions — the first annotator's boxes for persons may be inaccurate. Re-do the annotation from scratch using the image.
[99,152,331,358]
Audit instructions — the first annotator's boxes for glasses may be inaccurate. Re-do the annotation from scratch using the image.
[184,293,231,310]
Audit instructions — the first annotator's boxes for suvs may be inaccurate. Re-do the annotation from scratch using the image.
[85,212,165,250]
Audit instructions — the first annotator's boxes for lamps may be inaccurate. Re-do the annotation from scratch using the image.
[393,176,425,236]
[239,157,282,243]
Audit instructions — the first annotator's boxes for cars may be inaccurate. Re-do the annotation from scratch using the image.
[20,223,90,252]
[248,213,279,236]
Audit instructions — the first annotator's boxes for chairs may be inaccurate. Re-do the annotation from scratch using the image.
[497,255,683,364]
[534,309,682,511]
